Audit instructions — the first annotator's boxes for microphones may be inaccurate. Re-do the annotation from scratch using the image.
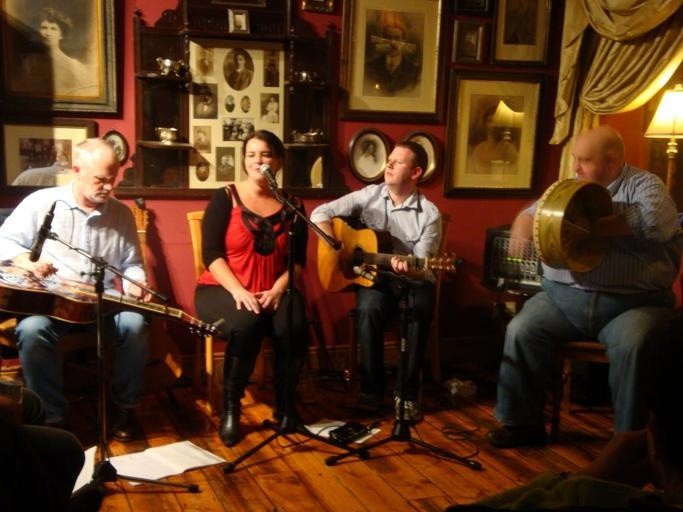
[352,265,380,283]
[260,164,277,188]
[29,211,54,262]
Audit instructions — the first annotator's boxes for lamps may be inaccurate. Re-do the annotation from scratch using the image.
[643,83,683,202]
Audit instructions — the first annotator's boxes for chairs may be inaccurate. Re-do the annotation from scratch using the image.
[347,211,453,396]
[551,336,610,424]
[57,207,165,392]
[187,211,310,417]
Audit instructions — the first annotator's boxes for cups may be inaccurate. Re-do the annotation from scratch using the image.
[155,126,178,144]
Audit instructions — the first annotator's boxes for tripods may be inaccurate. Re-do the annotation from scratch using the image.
[323,297,482,473]
[220,189,370,476]
[51,236,200,503]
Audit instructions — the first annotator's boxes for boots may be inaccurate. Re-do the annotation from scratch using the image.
[220,352,257,446]
[274,353,298,434]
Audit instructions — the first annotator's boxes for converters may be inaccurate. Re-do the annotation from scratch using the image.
[328,421,368,443]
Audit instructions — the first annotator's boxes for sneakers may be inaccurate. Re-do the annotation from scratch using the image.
[395,395,421,421]
[487,425,545,448]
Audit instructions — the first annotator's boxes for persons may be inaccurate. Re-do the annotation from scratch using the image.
[192,82,252,120]
[217,154,233,181]
[358,142,376,166]
[228,53,254,91]
[199,50,212,74]
[365,23,420,95]
[193,129,213,152]
[262,98,279,122]
[195,129,308,447]
[9,6,92,98]
[0,137,155,441]
[309,140,443,423]
[485,123,683,480]
[470,103,518,172]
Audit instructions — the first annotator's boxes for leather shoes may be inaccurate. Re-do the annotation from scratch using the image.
[109,407,134,442]
[71,485,102,512]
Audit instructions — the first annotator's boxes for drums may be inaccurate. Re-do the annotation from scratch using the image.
[532,178,612,270]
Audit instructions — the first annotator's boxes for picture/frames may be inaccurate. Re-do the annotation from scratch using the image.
[0,0,118,198]
[228,8,250,34]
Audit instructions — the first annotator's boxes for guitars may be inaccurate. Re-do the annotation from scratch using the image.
[317,215,463,292]
[134,195,182,396]
[0,266,222,336]
